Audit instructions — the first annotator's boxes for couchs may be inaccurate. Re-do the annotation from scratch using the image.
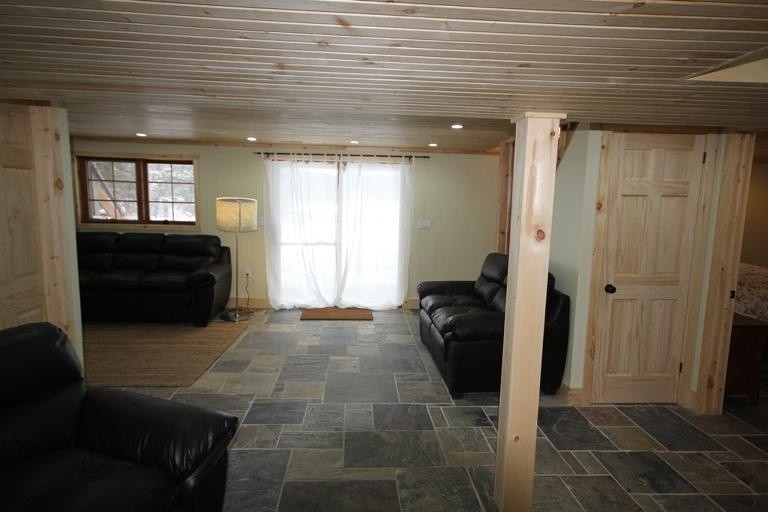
[415,252,571,400]
[77,230,232,327]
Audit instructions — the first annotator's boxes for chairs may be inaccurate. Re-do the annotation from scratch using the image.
[0,322,239,512]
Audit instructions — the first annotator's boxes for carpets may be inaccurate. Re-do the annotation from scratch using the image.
[301,305,374,321]
[81,308,268,388]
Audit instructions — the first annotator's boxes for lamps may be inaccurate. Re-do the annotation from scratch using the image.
[215,196,259,320]
